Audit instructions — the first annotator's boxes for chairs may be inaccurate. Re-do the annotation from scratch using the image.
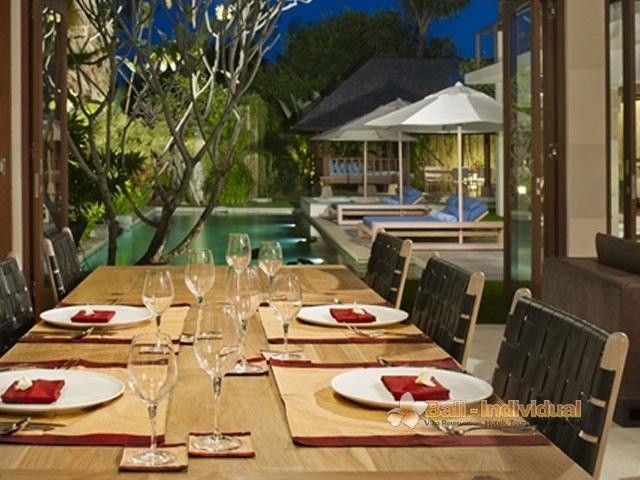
[0,226,84,361]
[326,184,504,251]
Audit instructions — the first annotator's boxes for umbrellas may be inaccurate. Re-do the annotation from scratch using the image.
[340,94,416,217]
[364,80,534,243]
[310,116,420,197]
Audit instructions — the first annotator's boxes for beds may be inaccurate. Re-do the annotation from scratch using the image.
[0,265,597,480]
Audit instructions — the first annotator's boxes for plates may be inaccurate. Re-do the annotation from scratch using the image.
[329,366,493,409]
[298,305,411,328]
[0,368,127,410]
[38,304,154,326]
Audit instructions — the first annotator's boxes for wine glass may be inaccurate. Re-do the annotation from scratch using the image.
[124,333,179,469]
[190,305,243,452]
[142,231,303,377]
